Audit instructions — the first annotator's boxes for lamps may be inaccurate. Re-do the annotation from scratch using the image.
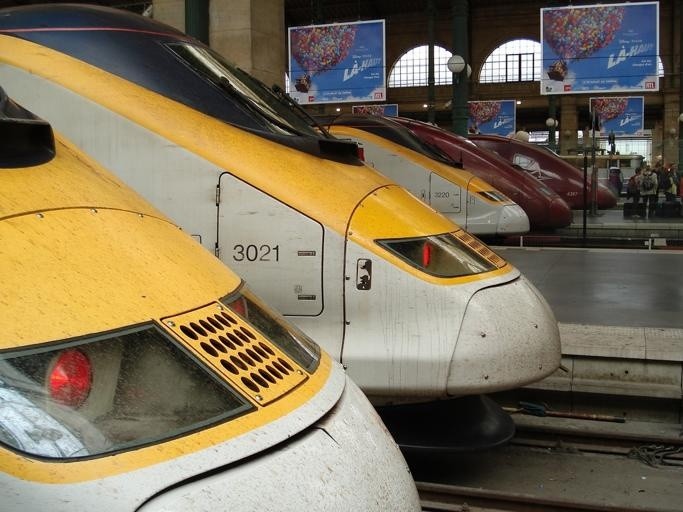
[447,55,465,85]
[669,128,676,141]
[467,64,472,79]
[555,119,559,128]
[546,116,554,128]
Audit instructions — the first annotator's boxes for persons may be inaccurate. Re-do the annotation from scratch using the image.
[620,156,682,221]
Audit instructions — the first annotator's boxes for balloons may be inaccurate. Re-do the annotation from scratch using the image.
[352,106,385,115]
[544,8,624,59]
[290,26,357,77]
[592,99,625,122]
[469,102,500,125]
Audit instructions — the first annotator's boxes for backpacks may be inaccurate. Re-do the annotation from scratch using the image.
[643,174,653,191]
[627,177,637,196]
[658,173,672,189]
